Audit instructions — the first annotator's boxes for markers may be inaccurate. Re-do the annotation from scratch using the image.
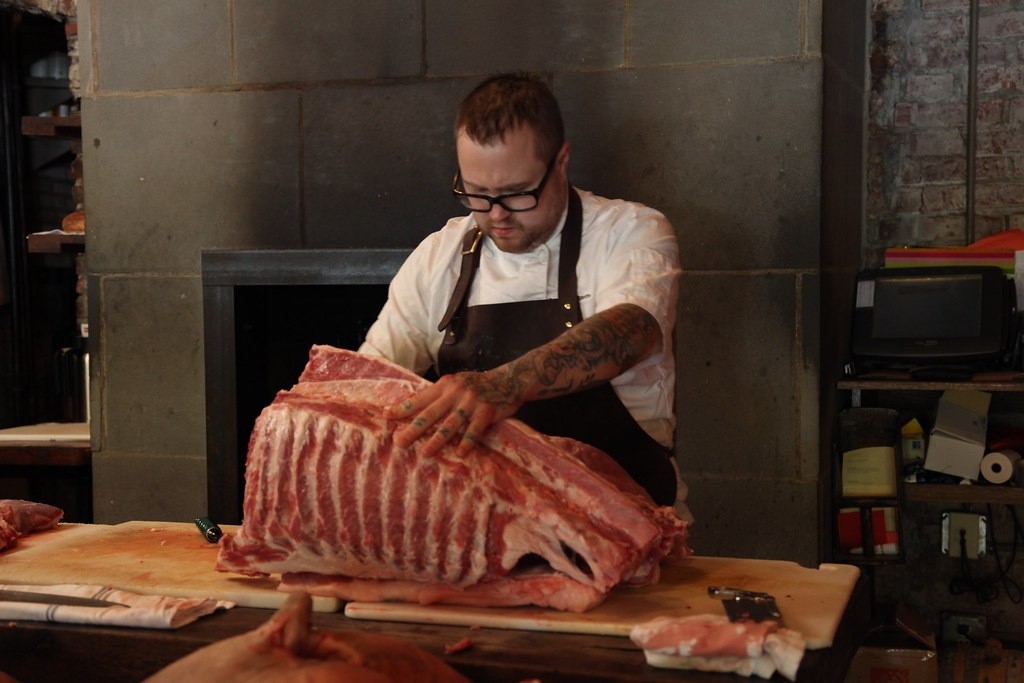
[708,586,775,601]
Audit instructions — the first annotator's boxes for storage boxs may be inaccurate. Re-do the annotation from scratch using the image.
[844,606,939,683]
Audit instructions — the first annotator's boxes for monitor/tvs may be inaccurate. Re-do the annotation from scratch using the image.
[848,265,1009,373]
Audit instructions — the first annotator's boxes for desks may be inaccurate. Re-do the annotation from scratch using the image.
[0,521,861,683]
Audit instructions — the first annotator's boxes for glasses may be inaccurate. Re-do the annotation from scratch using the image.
[452,155,555,212]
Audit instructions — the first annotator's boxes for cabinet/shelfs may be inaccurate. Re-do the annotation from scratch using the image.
[0,113,94,472]
[830,371,1024,566]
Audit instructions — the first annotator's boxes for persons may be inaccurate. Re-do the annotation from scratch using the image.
[356,68,700,532]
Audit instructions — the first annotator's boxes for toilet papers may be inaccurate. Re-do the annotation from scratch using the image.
[982,448,1021,485]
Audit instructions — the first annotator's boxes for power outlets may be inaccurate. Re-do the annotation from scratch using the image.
[941,612,987,645]
